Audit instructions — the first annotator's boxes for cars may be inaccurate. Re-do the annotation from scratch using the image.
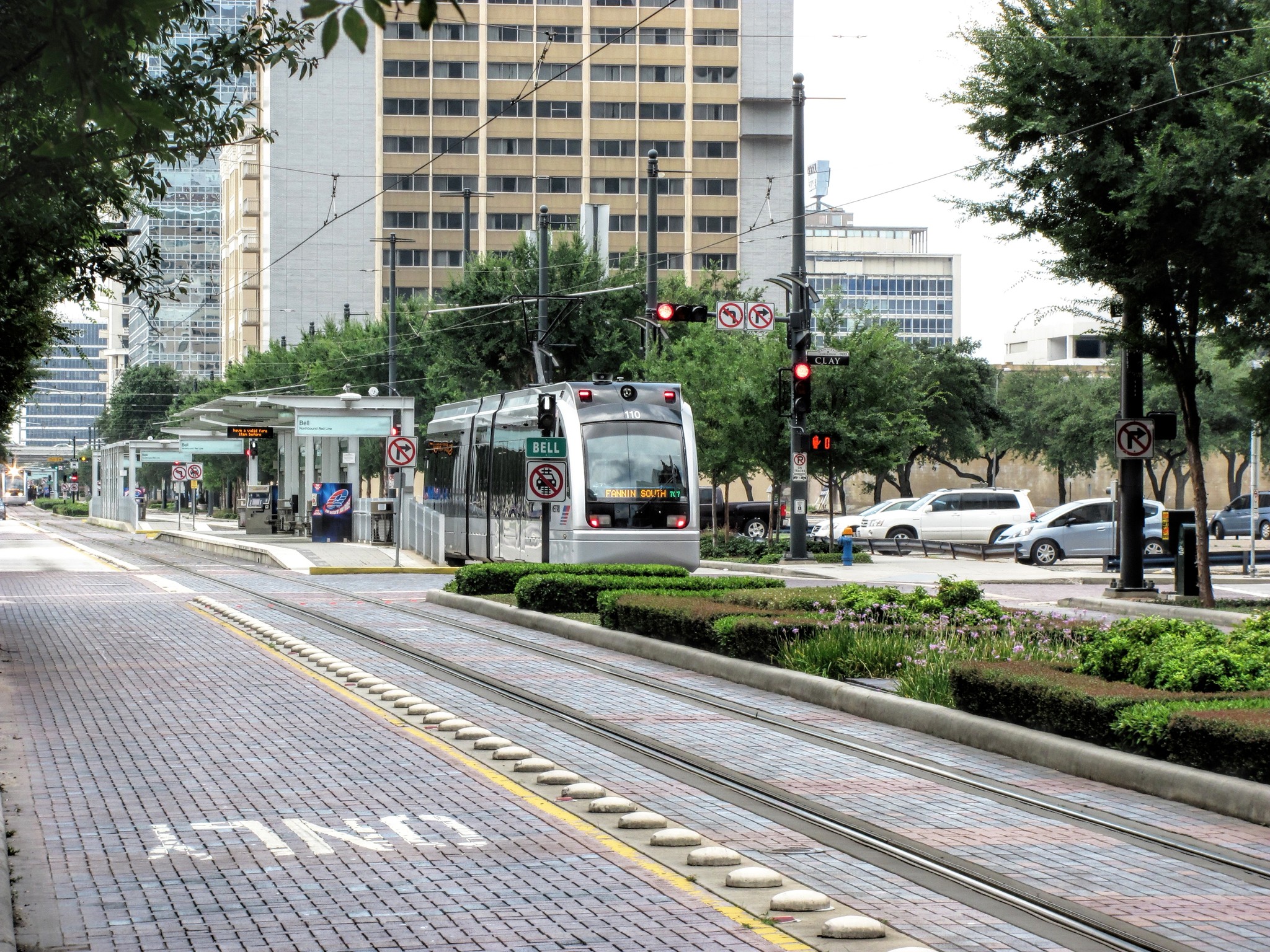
[1208,490,1270,541]
[809,497,957,557]
[992,495,1173,567]
[0,498,8,520]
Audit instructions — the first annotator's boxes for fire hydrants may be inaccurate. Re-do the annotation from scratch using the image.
[837,525,855,566]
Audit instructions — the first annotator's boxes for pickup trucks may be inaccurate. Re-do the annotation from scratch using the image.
[698,486,787,539]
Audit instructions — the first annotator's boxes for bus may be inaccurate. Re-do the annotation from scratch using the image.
[3,469,28,507]
[419,294,703,574]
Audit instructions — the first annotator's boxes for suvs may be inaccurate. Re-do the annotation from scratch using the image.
[854,486,1038,558]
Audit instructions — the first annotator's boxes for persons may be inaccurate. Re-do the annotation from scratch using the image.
[28,486,37,501]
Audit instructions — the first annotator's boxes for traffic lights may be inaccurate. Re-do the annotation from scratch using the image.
[250,440,258,456]
[809,432,830,451]
[656,303,707,323]
[72,470,77,481]
[79,457,91,461]
[51,463,58,469]
[791,361,812,414]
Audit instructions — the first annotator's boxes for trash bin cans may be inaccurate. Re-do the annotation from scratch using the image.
[135,497,146,521]
[236,498,246,529]
[277,499,292,533]
[370,501,394,547]
[1176,523,1209,596]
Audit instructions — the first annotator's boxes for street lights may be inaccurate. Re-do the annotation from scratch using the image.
[369,232,417,397]
[68,436,76,504]
[439,187,494,295]
